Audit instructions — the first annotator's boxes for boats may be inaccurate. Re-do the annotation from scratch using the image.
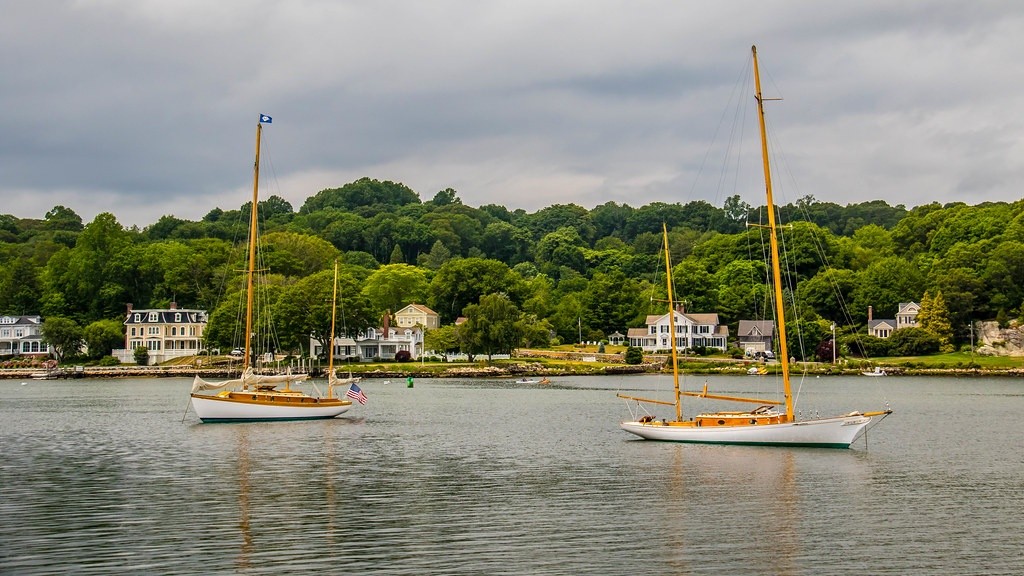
[861,366,888,376]
[30,362,63,380]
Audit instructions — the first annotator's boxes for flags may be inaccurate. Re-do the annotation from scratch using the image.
[346,384,367,405]
[260,114,272,123]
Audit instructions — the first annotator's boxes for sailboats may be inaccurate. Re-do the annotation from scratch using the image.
[617,45,895,451]
[189,112,360,420]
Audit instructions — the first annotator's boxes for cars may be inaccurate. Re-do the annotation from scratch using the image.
[231,347,245,356]
[755,352,769,361]
[752,351,775,359]
